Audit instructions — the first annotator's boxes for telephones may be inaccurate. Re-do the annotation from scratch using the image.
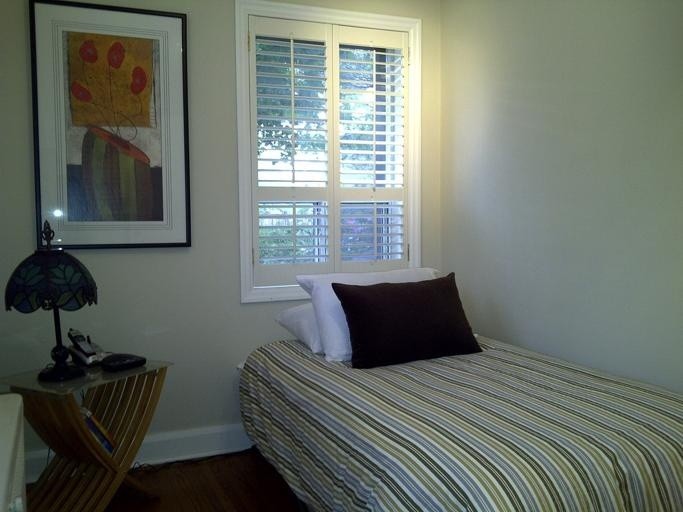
[67,327,112,367]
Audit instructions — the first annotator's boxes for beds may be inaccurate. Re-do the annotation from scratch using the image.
[241,330,682,512]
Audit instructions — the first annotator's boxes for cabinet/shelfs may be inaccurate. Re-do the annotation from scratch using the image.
[1,392,27,512]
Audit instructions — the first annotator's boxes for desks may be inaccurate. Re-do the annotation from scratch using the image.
[4,356,176,511]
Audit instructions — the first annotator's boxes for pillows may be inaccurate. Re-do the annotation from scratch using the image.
[331,271,484,369]
[275,301,324,356]
[295,268,441,364]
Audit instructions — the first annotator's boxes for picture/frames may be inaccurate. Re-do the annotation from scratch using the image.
[28,1,193,250]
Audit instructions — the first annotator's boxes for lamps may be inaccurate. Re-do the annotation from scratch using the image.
[4,220,100,384]
